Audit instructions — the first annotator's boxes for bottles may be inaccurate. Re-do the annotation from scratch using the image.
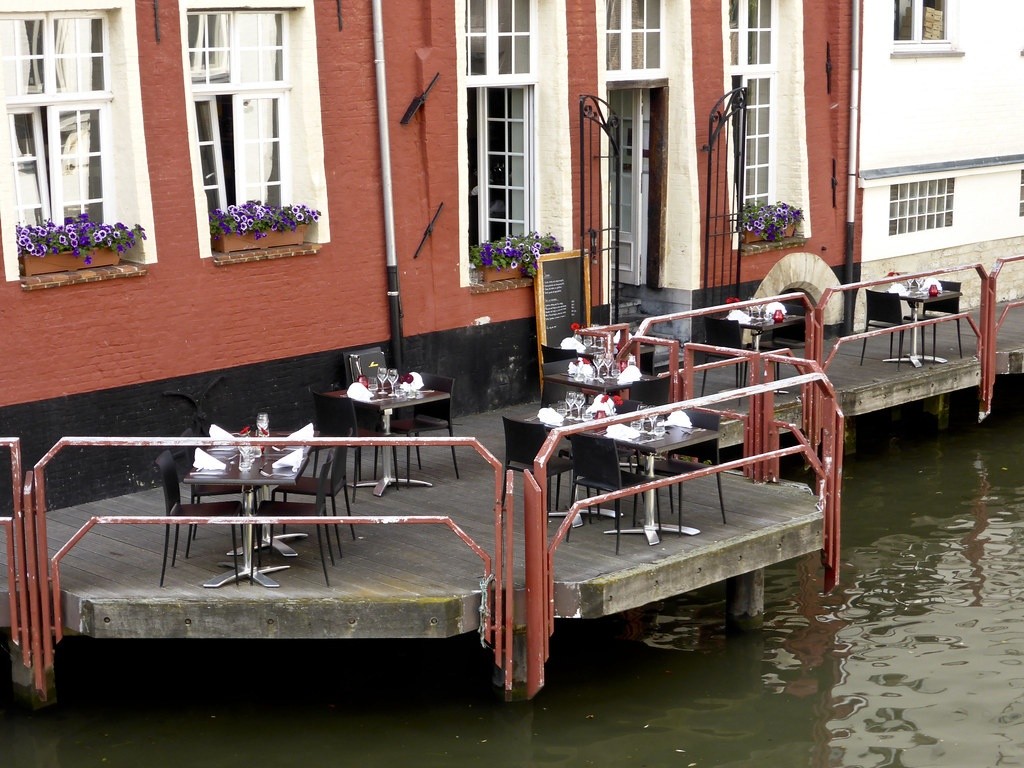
[238,413,268,472]
[566,356,592,382]
[395,382,423,401]
[594,410,608,431]
[359,374,368,389]
[620,353,636,372]
[377,366,398,397]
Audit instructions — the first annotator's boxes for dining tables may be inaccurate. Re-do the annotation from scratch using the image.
[340,387,451,497]
[184,428,319,589]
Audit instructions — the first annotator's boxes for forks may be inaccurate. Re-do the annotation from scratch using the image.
[272,446,297,452]
[260,471,293,478]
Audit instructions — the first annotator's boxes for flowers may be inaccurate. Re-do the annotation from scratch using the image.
[571,323,587,330]
[600,394,623,416]
[15,214,146,264]
[738,200,805,242]
[209,200,321,239]
[726,297,739,304]
[470,231,564,277]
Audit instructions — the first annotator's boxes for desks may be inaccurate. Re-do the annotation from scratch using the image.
[592,426,721,546]
[524,402,624,527]
[715,312,805,393]
[555,343,654,354]
[542,369,658,391]
[882,289,963,368]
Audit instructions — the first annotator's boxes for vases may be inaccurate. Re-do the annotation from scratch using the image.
[574,330,582,344]
[475,265,525,282]
[740,224,794,243]
[19,245,121,276]
[213,225,307,253]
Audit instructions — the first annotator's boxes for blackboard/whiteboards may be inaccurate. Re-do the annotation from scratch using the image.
[534,248,590,350]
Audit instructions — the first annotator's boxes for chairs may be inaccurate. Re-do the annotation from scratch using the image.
[500,398,733,553]
[860,289,913,372]
[181,427,251,558]
[701,303,808,398]
[538,335,674,473]
[374,371,459,486]
[250,449,335,588]
[270,427,355,558]
[904,281,963,365]
[155,450,244,587]
[312,390,399,502]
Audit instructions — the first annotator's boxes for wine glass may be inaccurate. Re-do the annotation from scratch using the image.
[637,404,658,435]
[744,295,772,324]
[581,323,613,350]
[593,351,614,380]
[905,269,924,297]
[565,391,585,420]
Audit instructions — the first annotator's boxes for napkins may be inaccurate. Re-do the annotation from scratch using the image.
[399,372,424,392]
[560,337,586,353]
[667,409,692,429]
[287,423,314,438]
[272,448,303,472]
[765,302,787,315]
[193,447,226,470]
[587,394,617,416]
[726,310,751,323]
[209,424,234,438]
[347,382,374,401]
[618,365,642,384]
[924,278,942,291]
[613,329,632,343]
[888,283,909,296]
[567,361,593,382]
[537,407,564,426]
[606,423,640,439]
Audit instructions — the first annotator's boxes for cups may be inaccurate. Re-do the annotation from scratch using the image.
[630,420,641,432]
[611,362,621,379]
[582,405,592,422]
[654,420,664,437]
[556,400,567,418]
[368,377,378,392]
